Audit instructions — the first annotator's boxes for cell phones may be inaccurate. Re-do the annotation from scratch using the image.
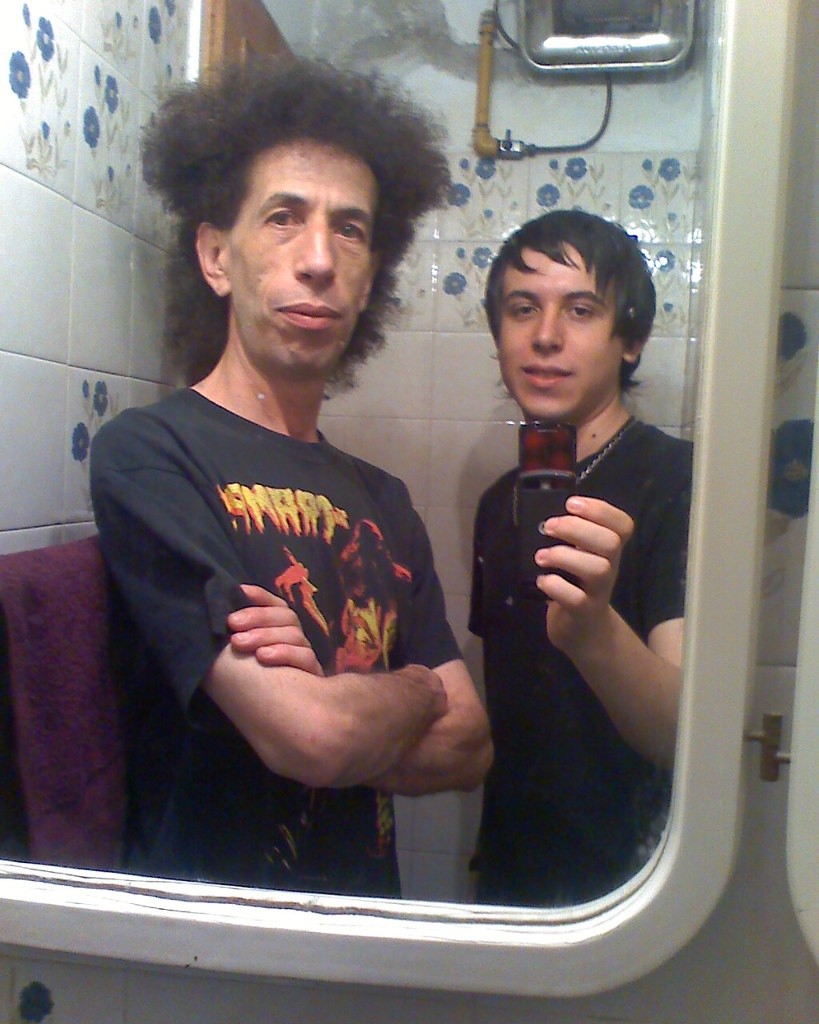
[516,421,578,590]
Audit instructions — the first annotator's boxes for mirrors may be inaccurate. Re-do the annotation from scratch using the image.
[0,1,798,999]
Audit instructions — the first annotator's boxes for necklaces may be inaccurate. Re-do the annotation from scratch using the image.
[511,416,642,529]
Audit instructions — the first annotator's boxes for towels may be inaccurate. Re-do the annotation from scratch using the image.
[0,533,129,871]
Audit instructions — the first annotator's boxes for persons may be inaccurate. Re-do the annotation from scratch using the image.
[461,207,693,913]
[88,63,495,902]
